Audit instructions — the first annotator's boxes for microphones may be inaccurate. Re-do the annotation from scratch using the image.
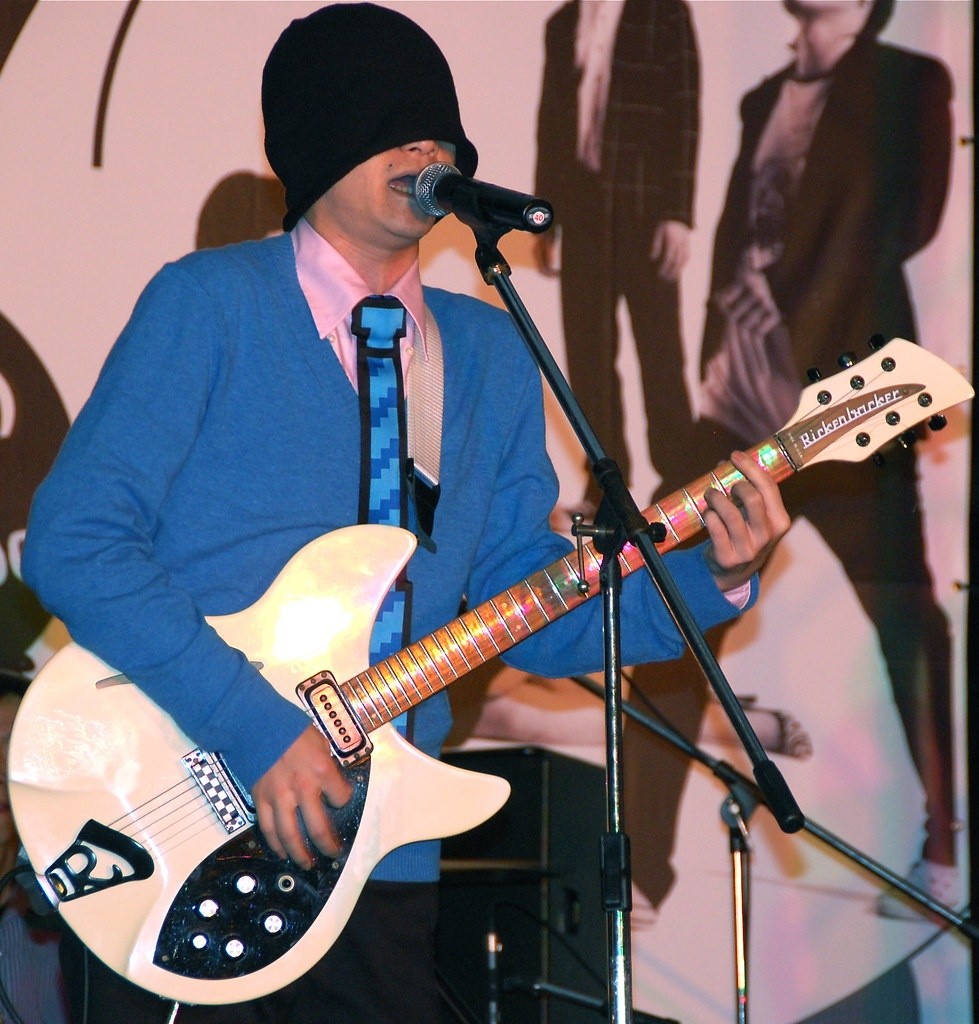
[411,161,555,234]
[483,911,501,1024]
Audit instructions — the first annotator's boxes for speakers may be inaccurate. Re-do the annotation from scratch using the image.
[431,744,615,1024]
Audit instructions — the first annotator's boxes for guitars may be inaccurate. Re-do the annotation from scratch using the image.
[3,332,975,1011]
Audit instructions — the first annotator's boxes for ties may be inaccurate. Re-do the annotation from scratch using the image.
[352,296,414,742]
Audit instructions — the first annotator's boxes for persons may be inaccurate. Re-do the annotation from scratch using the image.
[534,0,954,920]
[20,2,791,1023]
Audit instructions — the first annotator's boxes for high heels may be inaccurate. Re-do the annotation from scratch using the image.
[738,696,813,757]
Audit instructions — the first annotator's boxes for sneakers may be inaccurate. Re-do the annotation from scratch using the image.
[874,858,962,920]
[628,878,657,928]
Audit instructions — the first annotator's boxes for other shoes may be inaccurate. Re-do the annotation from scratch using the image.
[565,503,594,517]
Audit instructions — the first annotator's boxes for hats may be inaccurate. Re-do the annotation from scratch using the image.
[260,3,478,231]
